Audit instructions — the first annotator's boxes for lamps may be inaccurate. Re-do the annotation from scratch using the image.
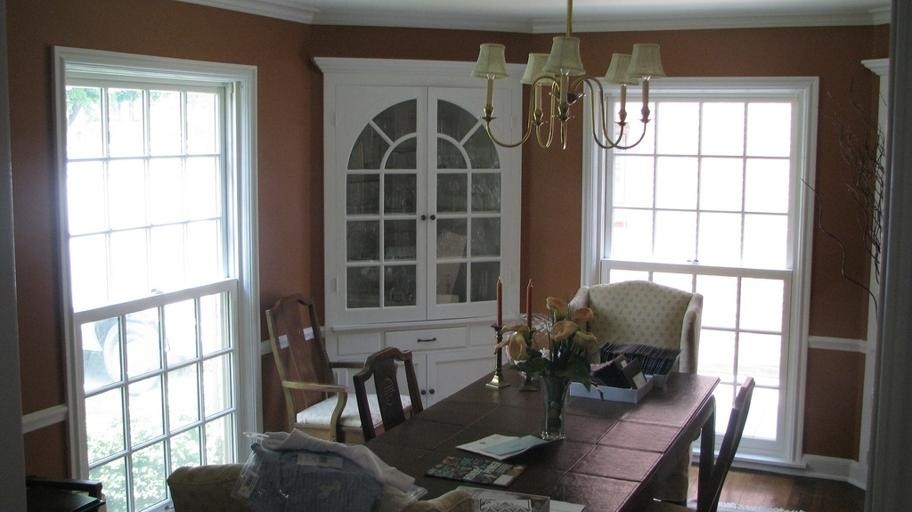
[474,0,665,152]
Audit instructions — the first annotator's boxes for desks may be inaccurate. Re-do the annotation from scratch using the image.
[356,356,721,512]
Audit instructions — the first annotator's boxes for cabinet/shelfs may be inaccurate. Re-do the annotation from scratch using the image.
[317,313,522,410]
[310,56,529,328]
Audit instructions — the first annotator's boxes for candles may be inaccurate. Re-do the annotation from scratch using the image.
[524,277,536,330]
[495,273,505,323]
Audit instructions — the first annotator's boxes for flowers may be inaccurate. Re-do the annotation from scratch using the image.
[498,294,610,438]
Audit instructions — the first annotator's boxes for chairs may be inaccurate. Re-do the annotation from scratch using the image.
[164,459,478,511]
[562,279,704,505]
[353,345,426,444]
[643,373,758,512]
[264,294,412,444]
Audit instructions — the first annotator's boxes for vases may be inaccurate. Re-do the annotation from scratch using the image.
[540,371,569,444]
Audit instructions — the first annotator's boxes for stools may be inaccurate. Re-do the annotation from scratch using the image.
[26,469,107,512]
